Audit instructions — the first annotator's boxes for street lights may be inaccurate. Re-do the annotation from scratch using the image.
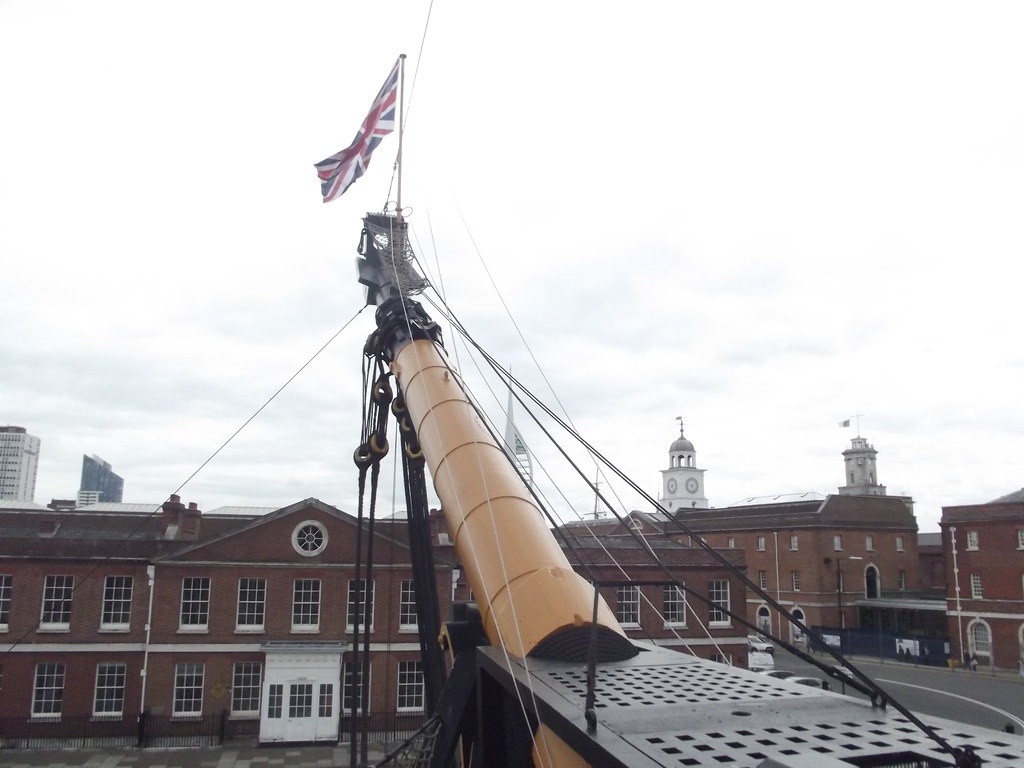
[837,556,864,693]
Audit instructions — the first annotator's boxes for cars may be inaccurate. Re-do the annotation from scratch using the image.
[747,635,774,653]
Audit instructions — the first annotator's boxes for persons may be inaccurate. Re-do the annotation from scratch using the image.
[807,636,816,654]
[898,644,911,662]
[963,649,970,671]
[971,651,977,671]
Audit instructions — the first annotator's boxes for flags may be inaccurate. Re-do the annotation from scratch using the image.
[314,57,399,203]
